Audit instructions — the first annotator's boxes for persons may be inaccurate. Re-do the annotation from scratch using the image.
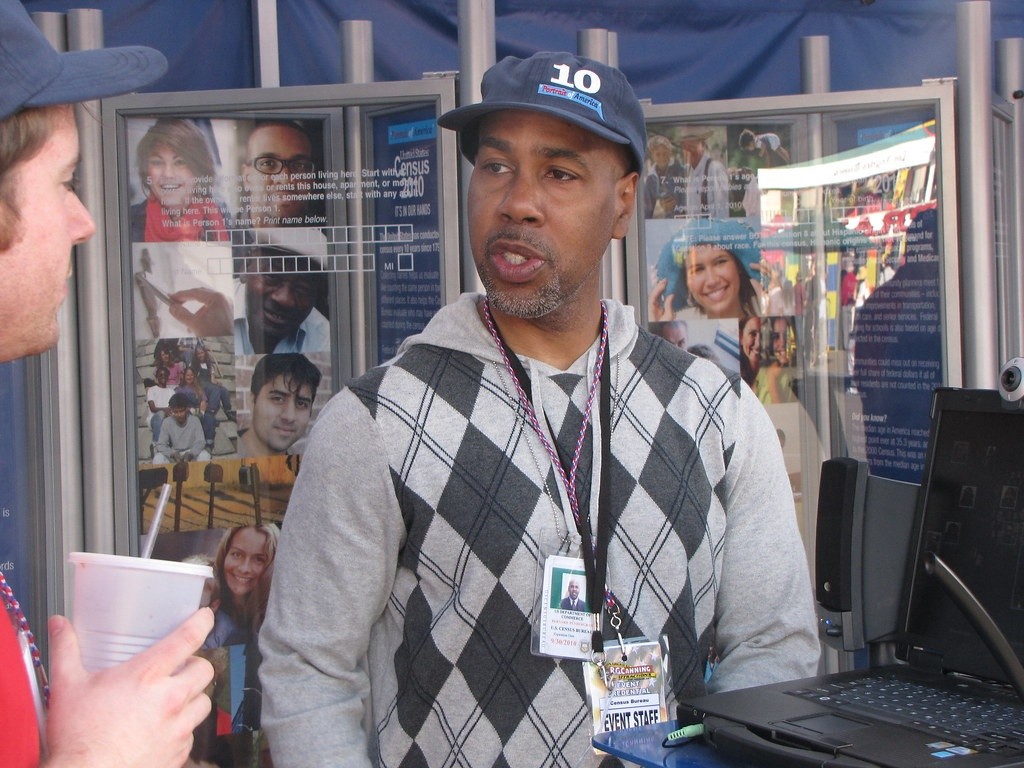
[0,0,217,767]
[730,127,791,217]
[254,48,823,768]
[230,116,316,228]
[642,220,798,406]
[169,286,235,337]
[126,115,251,244]
[562,578,587,612]
[200,524,281,651]
[236,352,324,458]
[135,339,236,487]
[644,124,729,220]
[230,229,331,353]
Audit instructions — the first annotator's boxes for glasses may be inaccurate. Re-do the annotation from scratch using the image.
[244,155,318,178]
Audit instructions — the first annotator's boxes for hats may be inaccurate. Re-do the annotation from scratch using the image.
[656,216,771,311]
[0,0,169,119]
[436,50,646,180]
[248,226,328,269]
[672,121,715,143]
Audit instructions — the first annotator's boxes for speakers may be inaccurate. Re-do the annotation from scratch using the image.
[815,456,923,652]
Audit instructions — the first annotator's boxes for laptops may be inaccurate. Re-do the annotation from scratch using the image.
[676,386,1024,768]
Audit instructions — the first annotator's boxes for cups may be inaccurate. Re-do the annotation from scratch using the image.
[67,551,215,673]
